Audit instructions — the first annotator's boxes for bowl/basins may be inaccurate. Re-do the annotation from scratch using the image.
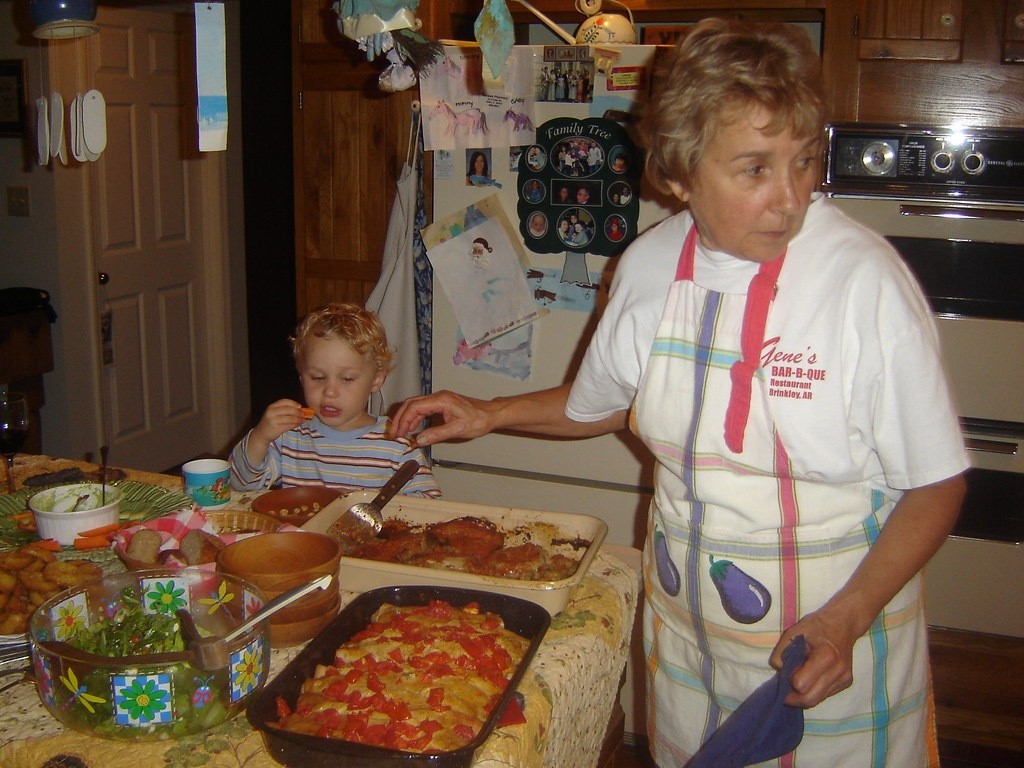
[251,484,342,528]
[214,533,343,651]
[110,509,300,572]
[26,571,270,743]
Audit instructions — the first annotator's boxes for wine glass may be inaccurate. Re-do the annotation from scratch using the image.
[0,392,28,494]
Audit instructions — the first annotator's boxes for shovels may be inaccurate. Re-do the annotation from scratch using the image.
[327,459,420,544]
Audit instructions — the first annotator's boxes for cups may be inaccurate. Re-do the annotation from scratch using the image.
[181,458,232,510]
[29,483,125,546]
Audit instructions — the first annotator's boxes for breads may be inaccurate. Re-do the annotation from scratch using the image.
[126,529,225,565]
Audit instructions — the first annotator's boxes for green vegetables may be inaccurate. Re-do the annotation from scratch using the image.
[58,588,230,741]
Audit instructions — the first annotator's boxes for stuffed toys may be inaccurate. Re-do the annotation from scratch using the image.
[329,0,445,93]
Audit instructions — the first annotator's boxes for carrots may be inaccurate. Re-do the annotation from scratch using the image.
[299,408,314,419]
[8,510,142,551]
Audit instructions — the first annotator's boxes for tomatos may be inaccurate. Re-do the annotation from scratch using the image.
[264,603,511,753]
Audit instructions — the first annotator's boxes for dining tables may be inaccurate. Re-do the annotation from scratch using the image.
[0,443,646,767]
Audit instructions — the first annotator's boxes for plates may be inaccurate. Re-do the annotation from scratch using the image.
[245,583,552,768]
[297,491,610,617]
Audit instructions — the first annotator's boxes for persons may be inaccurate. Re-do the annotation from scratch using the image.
[608,219,624,240]
[528,147,546,169]
[612,152,626,172]
[555,185,592,204]
[559,214,591,246]
[526,181,543,203]
[539,66,591,103]
[530,214,546,235]
[559,140,603,177]
[466,152,489,185]
[227,303,442,500]
[386,16,973,768]
[612,187,631,205]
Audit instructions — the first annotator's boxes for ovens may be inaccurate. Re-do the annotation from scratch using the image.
[822,191,1024,642]
